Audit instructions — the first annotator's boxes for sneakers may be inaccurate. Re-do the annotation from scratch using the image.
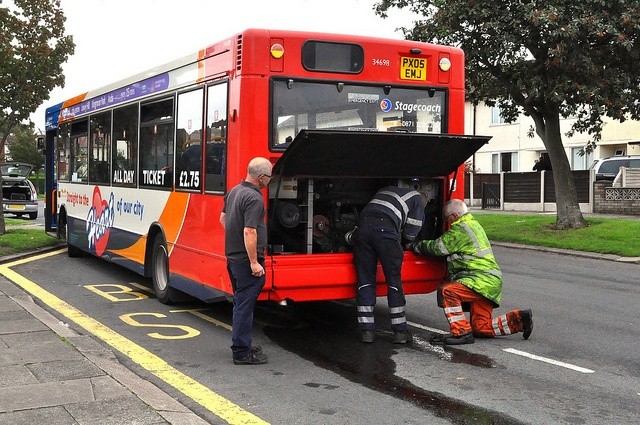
[251,345,264,354]
[519,309,533,340]
[445,333,475,345]
[392,331,413,343]
[358,330,375,342]
[233,351,268,365]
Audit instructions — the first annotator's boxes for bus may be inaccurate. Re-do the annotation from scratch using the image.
[36,27,494,306]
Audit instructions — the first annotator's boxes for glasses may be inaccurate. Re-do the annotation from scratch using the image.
[258,173,273,178]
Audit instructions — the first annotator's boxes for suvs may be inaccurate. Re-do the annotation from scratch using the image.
[1,161,37,219]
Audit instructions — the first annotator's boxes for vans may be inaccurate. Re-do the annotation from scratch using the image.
[588,154,639,176]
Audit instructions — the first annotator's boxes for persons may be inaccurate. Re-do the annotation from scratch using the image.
[533,157,547,172]
[350,186,428,343]
[404,199,534,345]
[219,157,273,366]
